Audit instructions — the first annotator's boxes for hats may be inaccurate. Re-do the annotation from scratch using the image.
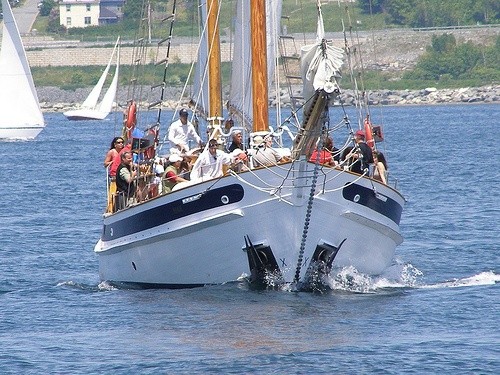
[252,136,265,146]
[180,108,188,116]
[155,165,165,174]
[168,154,183,162]
[356,130,365,136]
[232,129,241,138]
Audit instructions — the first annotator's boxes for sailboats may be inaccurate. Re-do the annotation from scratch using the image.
[0,0,45,141]
[63,37,121,121]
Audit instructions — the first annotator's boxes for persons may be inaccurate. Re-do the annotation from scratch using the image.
[349,130,374,177]
[162,154,190,195]
[167,108,206,156]
[190,139,248,182]
[372,151,388,185]
[104,137,125,213]
[252,134,292,169]
[113,150,138,212]
[309,137,335,167]
[228,130,248,155]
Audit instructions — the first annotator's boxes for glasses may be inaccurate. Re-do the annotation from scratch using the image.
[209,143,218,147]
[115,141,124,144]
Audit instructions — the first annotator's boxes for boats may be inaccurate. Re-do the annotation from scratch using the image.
[93,0,405,291]
